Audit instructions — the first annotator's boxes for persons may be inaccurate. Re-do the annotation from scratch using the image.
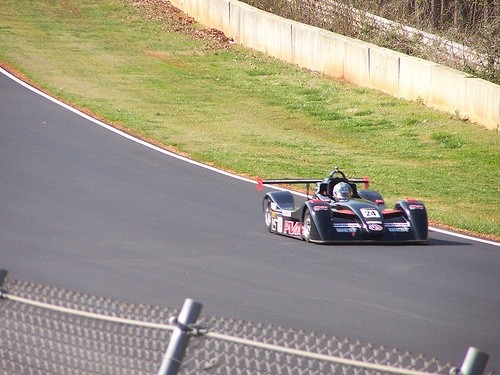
[330,182,352,203]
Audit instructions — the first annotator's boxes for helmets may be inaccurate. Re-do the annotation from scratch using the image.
[333,182,353,201]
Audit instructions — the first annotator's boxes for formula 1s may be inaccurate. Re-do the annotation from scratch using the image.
[256,168,431,245]
